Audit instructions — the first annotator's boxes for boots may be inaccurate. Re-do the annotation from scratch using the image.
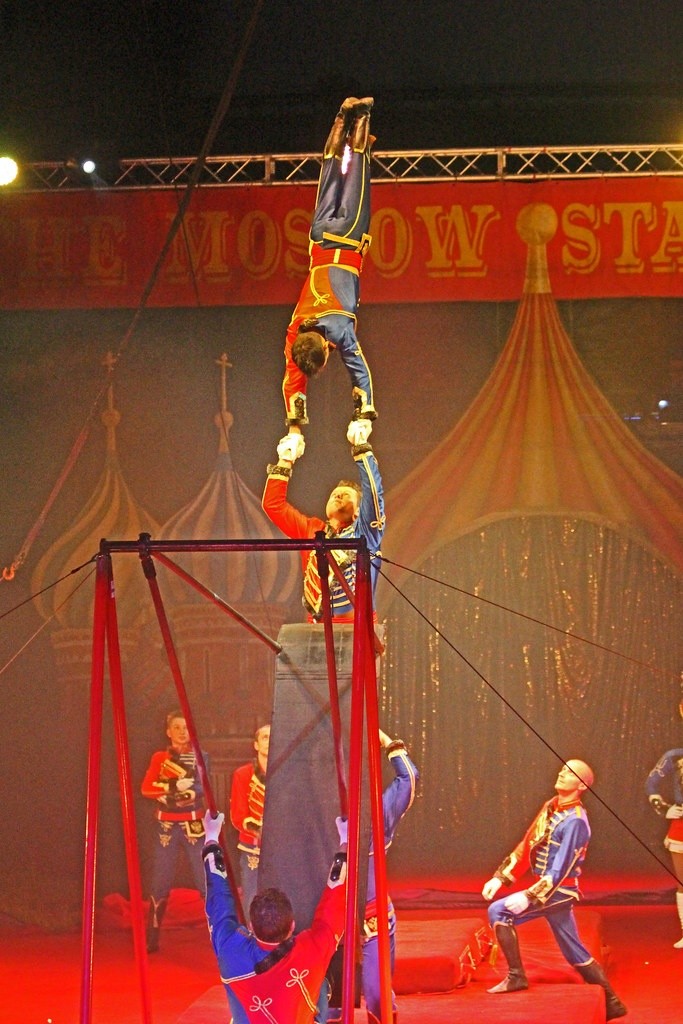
[673,892,683,948]
[351,96,376,153]
[146,895,168,953]
[276,433,303,464]
[487,921,529,993]
[574,958,627,1021]
[322,97,359,161]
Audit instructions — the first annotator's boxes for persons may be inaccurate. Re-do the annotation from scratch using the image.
[360,729,419,1024]
[482,759,627,1022]
[646,747,682,949]
[229,722,270,928]
[140,712,210,956]
[277,96,378,445]
[257,421,384,623]
[199,810,347,1024]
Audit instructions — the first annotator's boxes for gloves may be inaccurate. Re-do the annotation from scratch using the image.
[482,877,502,900]
[505,894,531,915]
[351,422,367,446]
[278,439,305,461]
[335,817,348,846]
[348,417,372,443]
[203,809,225,845]
[181,790,195,806]
[665,804,683,819]
[176,777,194,791]
